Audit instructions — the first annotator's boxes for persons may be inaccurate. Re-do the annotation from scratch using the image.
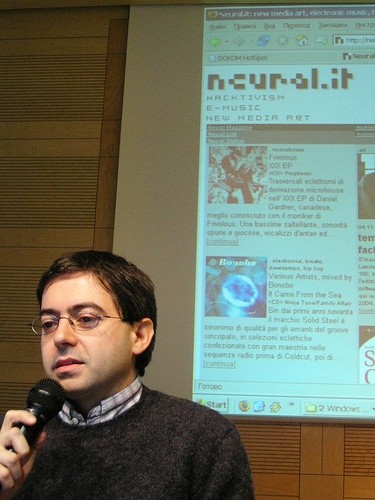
[0,250,254,500]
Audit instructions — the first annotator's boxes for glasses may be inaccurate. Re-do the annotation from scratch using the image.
[30,308,124,336]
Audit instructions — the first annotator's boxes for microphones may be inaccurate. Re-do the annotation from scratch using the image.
[0,378,67,492]
